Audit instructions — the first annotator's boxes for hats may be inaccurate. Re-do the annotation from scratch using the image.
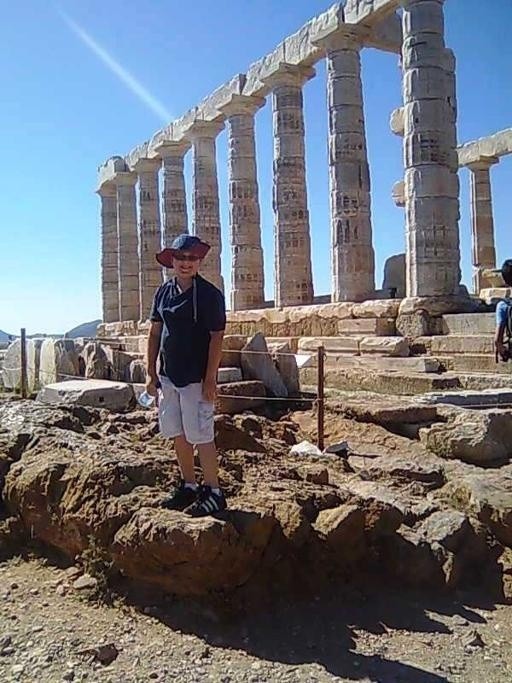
[155,234,211,268]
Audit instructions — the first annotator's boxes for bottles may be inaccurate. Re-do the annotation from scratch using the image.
[138,381,161,406]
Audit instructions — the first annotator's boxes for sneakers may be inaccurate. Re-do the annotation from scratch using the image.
[184,485,227,517]
[159,479,202,507]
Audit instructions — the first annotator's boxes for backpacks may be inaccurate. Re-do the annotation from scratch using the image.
[502,297,512,339]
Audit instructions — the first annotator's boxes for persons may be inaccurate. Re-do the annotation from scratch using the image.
[492,258,512,364]
[144,232,227,519]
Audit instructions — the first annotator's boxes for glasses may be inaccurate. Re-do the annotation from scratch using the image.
[172,252,200,262]
